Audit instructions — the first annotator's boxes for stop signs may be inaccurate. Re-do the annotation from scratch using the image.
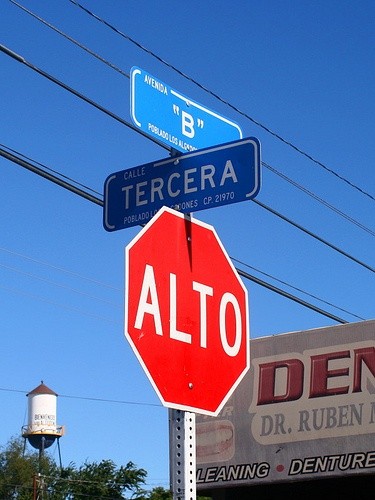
[123,205,250,417]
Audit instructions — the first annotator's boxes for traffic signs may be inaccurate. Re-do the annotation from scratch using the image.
[101,136,262,233]
[128,66,242,154]
